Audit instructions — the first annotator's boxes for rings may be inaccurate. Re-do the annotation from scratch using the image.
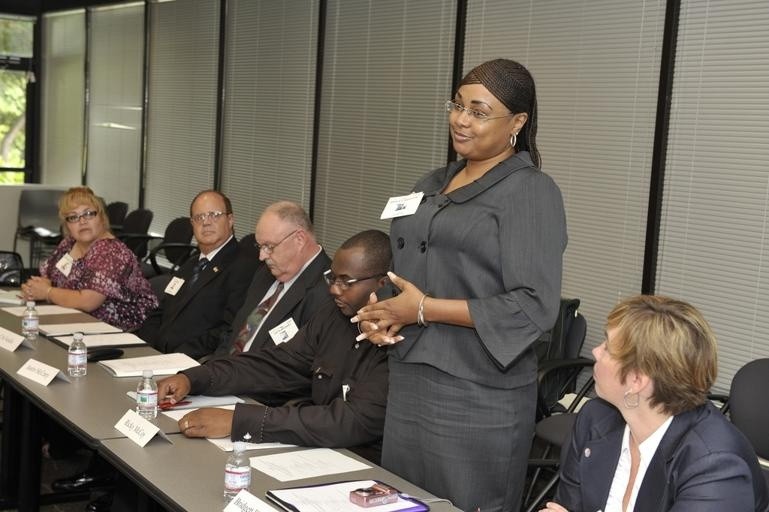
[377,343,382,348]
[183,420,189,427]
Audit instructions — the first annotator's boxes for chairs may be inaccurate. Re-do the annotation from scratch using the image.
[1,185,198,280]
[707,360,768,466]
[522,297,598,511]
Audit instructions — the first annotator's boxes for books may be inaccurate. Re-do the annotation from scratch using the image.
[97,352,201,378]
[39,322,124,337]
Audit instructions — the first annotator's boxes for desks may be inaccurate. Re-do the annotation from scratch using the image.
[0,289,458,512]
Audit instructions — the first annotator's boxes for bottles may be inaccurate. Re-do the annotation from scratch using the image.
[68,334,88,377]
[21,301,40,340]
[137,369,158,420]
[223,442,251,504]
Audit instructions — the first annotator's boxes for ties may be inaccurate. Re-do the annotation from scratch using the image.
[229,282,284,354]
[184,258,210,287]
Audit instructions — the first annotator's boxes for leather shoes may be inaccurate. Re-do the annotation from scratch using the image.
[86,493,113,512]
[51,470,95,493]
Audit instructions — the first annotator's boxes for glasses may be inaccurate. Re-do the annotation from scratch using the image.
[446,99,514,121]
[64,211,97,223]
[322,269,387,290]
[190,211,229,223]
[254,230,300,254]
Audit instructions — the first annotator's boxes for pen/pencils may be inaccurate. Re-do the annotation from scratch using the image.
[159,401,192,409]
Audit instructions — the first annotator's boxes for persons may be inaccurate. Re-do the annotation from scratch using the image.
[50,200,332,511]
[155,226,392,468]
[20,186,160,332]
[133,189,261,360]
[350,58,568,511]
[536,295,769,511]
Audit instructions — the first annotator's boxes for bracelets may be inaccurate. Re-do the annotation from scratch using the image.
[47,287,52,303]
[357,320,367,340]
[418,293,431,329]
